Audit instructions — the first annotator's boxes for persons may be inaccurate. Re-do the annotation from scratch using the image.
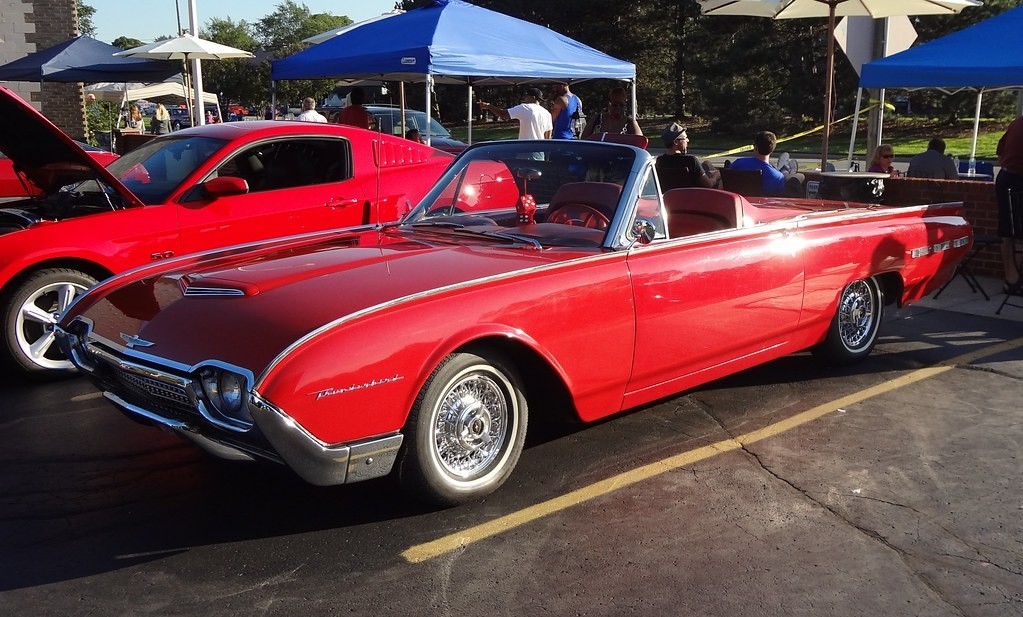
[580,87,643,139]
[265,106,272,120]
[296,97,327,122]
[126,105,145,134]
[995,117,1023,296]
[406,129,426,144]
[338,88,369,130]
[731,131,798,196]
[85,94,100,120]
[868,144,899,176]
[907,137,958,179]
[206,111,214,124]
[476,83,582,140]
[655,122,730,194]
[151,103,173,135]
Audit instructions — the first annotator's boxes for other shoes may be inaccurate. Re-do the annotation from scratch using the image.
[788,159,798,173]
[777,152,790,173]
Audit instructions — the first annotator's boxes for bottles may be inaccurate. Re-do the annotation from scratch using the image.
[953,156,960,174]
[967,154,976,176]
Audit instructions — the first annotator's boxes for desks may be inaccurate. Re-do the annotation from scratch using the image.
[820,171,890,203]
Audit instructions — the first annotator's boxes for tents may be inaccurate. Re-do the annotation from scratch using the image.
[846,4,1023,173]
[0,35,223,153]
[270,0,637,147]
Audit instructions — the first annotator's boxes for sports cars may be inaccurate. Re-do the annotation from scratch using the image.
[45,134,978,510]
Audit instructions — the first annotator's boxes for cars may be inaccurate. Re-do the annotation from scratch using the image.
[0,140,152,206]
[314,102,469,156]
[165,107,198,132]
[0,82,523,386]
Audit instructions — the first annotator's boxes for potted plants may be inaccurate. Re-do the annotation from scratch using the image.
[86,99,121,154]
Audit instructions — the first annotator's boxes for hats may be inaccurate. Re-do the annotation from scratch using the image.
[661,122,687,148]
[527,88,545,102]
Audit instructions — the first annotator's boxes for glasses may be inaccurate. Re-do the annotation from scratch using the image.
[91,99,96,100]
[881,154,894,158]
[611,101,626,108]
[676,136,687,141]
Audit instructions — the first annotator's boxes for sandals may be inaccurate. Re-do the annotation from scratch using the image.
[1004,278,1023,296]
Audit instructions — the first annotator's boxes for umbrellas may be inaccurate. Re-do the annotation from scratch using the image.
[113,31,255,127]
[303,7,407,139]
[697,0,984,172]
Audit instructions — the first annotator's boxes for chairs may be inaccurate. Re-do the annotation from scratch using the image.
[720,167,767,197]
[392,117,411,135]
[653,153,698,193]
[927,180,992,300]
[996,188,1022,317]
[655,187,757,240]
[540,182,624,231]
